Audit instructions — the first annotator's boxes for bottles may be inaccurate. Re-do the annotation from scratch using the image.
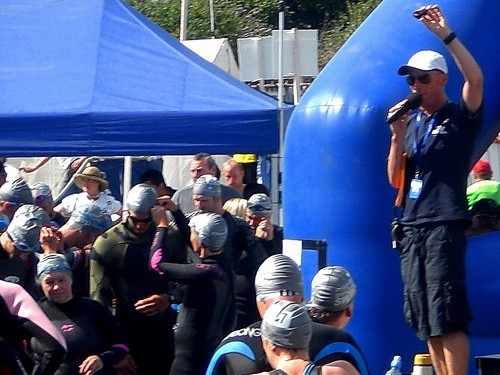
[384,355,402,375]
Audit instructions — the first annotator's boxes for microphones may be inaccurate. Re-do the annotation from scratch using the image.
[385,91,423,124]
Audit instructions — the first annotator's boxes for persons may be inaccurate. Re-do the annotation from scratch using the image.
[466,160,500,210]
[388,5,484,375]
[0,152,282,375]
[205,254,370,375]
[465,198,500,236]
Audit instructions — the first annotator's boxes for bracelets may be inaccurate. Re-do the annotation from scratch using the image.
[443,32,456,45]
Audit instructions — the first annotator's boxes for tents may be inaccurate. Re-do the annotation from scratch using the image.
[0,0,294,227]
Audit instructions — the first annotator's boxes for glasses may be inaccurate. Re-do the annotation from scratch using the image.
[16,243,39,252]
[247,202,272,211]
[35,195,47,202]
[407,73,440,85]
[130,216,152,223]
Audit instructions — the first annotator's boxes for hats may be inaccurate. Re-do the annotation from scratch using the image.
[397,50,449,75]
[246,193,272,216]
[126,184,160,215]
[68,204,112,235]
[261,300,312,348]
[36,253,73,276]
[74,167,108,193]
[255,254,304,301]
[472,160,491,172]
[30,182,53,203]
[1,177,35,207]
[311,266,357,311]
[7,204,51,252]
[193,174,221,198]
[189,212,227,249]
